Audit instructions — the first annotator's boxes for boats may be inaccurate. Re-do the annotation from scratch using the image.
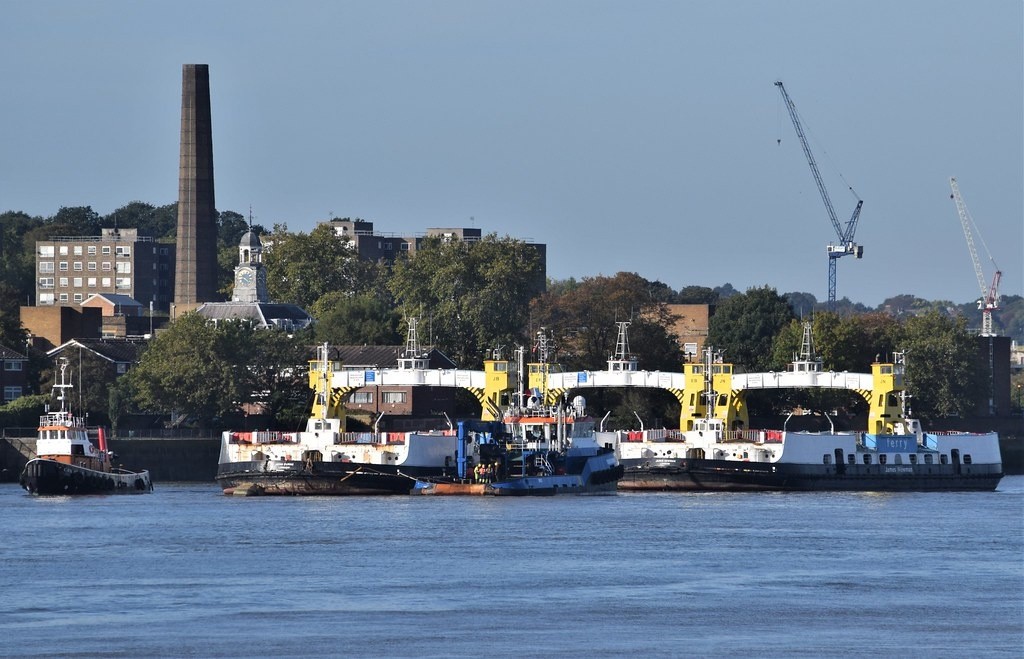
[394,343,625,497]
[615,345,1007,493]
[19,363,154,498]
[217,342,480,498]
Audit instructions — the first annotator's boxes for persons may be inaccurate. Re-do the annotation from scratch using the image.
[473,461,502,485]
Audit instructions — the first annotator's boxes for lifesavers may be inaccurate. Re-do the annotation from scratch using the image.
[89,445,95,453]
[138,478,146,491]
[118,481,122,489]
[71,472,115,491]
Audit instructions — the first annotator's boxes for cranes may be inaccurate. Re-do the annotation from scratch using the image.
[949,175,1003,338]
[775,80,864,314]
